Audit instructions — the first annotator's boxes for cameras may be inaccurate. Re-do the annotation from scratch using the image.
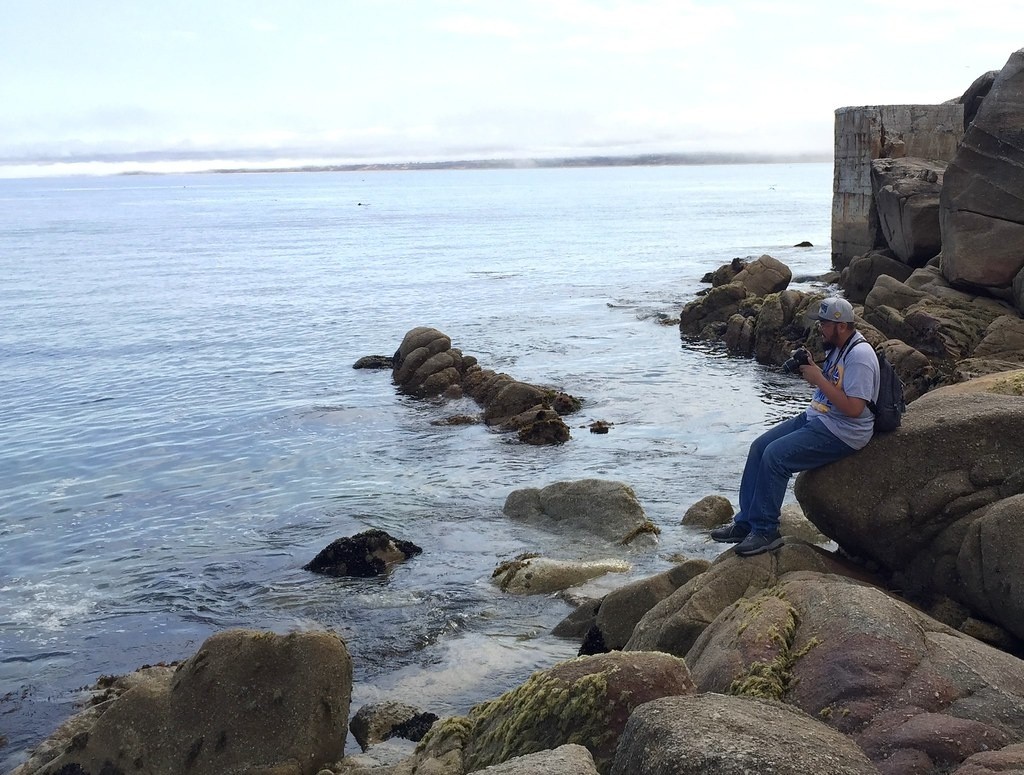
[782,348,809,376]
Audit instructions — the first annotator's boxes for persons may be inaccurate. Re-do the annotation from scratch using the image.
[712,297,881,552]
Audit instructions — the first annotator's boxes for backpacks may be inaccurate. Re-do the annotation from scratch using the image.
[842,338,906,432]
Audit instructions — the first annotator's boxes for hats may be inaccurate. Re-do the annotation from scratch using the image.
[806,297,855,322]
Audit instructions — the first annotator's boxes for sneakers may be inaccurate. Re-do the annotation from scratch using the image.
[734,530,786,556]
[709,523,750,542]
